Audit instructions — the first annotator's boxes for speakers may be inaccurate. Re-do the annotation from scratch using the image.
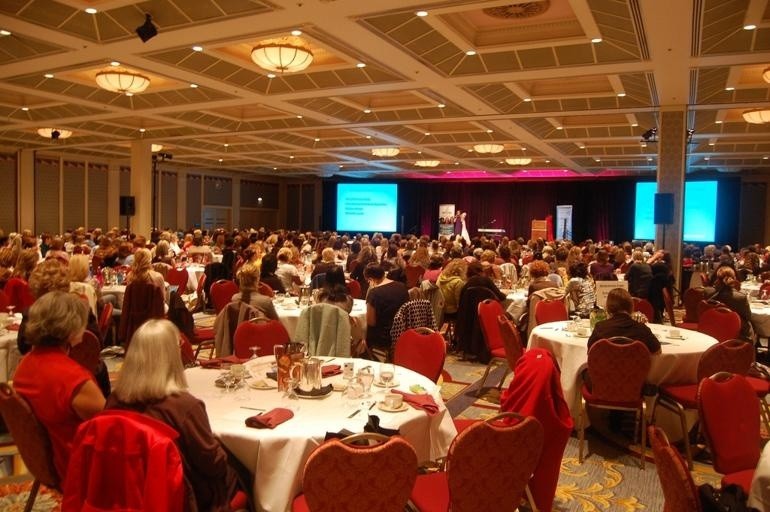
[120,195,135,216]
[654,193,674,225]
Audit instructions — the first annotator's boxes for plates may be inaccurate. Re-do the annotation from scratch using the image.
[667,336,683,339]
[378,401,410,413]
[246,377,279,391]
[372,376,400,389]
[281,385,334,399]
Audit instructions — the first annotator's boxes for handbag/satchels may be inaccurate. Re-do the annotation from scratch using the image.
[698,484,760,512]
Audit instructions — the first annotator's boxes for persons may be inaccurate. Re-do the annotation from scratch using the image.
[1,226,769,511]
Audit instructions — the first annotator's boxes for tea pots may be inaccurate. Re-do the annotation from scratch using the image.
[288,353,326,392]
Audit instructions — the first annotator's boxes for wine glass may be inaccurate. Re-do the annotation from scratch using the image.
[281,378,300,413]
[221,346,262,398]
[379,363,395,394]
[746,274,763,288]
[761,290,768,300]
[568,311,583,330]
[357,369,374,399]
[6,305,15,317]
[272,288,319,310]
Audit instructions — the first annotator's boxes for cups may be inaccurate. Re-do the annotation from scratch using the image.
[578,328,587,336]
[669,327,681,337]
[384,393,403,409]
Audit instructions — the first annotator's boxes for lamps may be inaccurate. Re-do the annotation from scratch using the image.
[250,43,314,73]
[415,161,440,167]
[762,68,770,83]
[37,128,73,139]
[742,109,770,124]
[504,159,532,166]
[151,144,163,152]
[95,71,150,93]
[472,144,504,154]
[371,148,400,157]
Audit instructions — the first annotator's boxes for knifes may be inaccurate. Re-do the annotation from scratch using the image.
[239,405,266,413]
[324,356,337,364]
